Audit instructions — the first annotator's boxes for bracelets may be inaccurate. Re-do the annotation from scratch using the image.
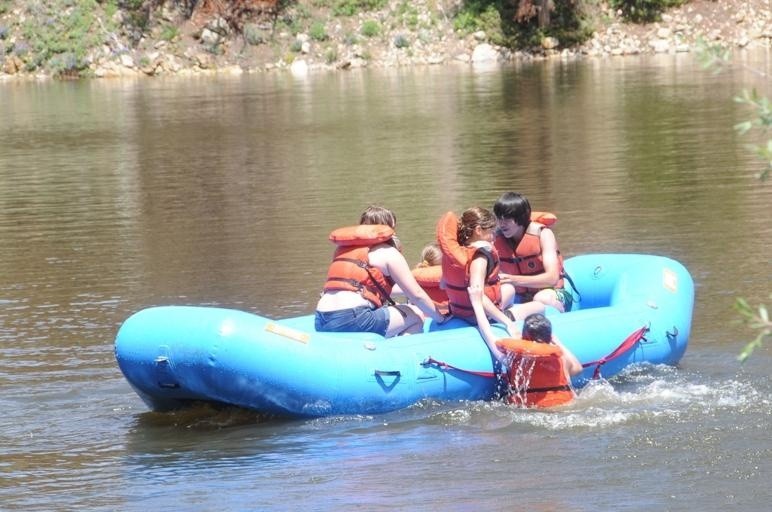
[437,316,445,325]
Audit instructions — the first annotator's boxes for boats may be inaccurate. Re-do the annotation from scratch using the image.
[107,254,698,419]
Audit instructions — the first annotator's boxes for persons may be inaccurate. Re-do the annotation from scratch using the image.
[411,244,450,317]
[439,205,546,326]
[314,205,453,337]
[490,192,574,315]
[467,280,583,409]
